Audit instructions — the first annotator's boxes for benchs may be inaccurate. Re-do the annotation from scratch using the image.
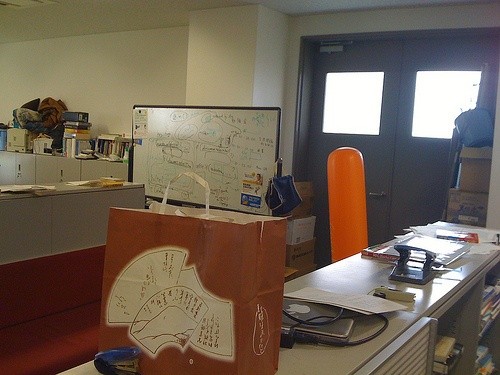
[0,244,104,375]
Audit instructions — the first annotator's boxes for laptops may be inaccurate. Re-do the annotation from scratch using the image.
[282,298,359,347]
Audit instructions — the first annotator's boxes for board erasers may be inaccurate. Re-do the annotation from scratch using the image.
[181,203,197,208]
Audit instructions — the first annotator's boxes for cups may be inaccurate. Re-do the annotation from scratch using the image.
[0,123,8,151]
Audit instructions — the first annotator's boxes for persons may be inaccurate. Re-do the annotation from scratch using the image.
[256,174,262,185]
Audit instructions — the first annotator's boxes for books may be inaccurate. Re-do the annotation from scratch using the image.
[62,112,92,158]
[474,345,496,375]
[480,276,500,337]
[433,335,464,375]
[362,244,400,260]
[95,137,123,157]
[436,229,479,243]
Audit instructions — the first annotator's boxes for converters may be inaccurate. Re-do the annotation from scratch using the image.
[280,327,296,349]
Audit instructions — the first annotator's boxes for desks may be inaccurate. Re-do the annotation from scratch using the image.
[0,178,146,265]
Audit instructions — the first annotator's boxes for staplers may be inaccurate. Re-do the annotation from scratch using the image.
[93,347,140,375]
[376,285,417,302]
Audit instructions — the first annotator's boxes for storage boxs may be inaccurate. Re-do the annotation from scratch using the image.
[33,138,54,155]
[0,128,7,151]
[286,215,316,245]
[446,188,490,227]
[6,128,38,153]
[279,181,316,221]
[285,236,316,278]
[458,146,493,193]
[62,111,89,123]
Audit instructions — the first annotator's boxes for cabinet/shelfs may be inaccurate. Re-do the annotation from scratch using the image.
[273,219,500,375]
[80,159,129,182]
[35,154,81,185]
[0,151,35,184]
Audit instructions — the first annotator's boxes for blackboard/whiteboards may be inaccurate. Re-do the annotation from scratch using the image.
[129,104,282,216]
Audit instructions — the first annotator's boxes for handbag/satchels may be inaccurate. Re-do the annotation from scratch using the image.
[265,156,303,215]
